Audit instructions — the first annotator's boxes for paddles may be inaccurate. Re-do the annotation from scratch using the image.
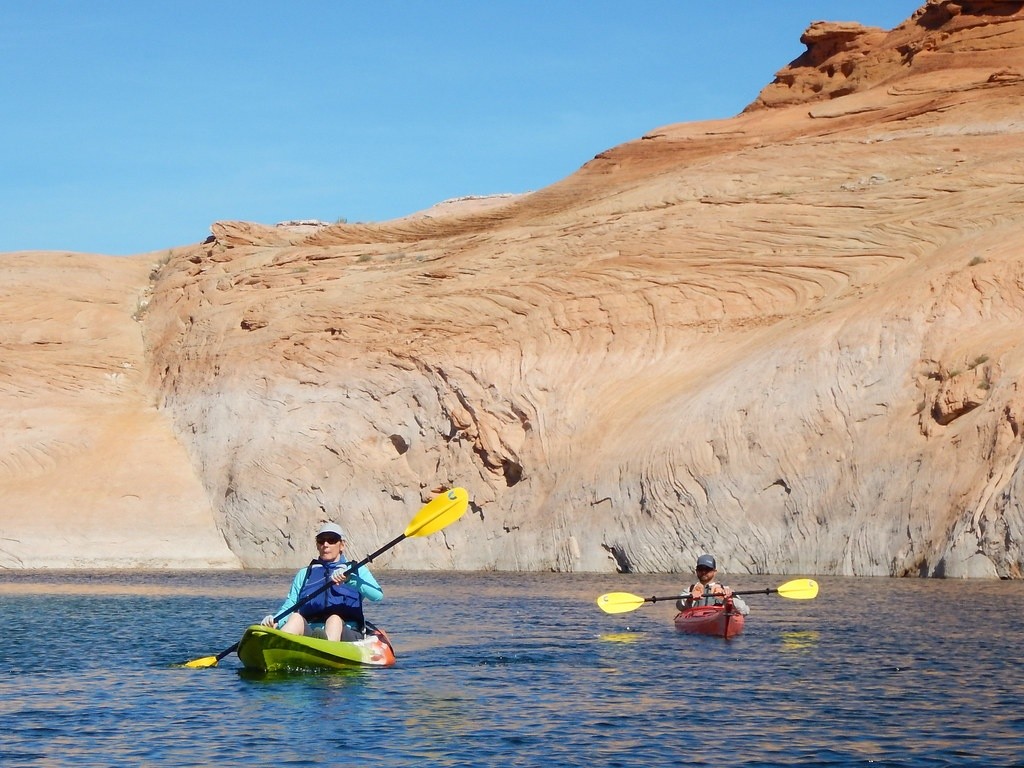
[595,577,821,616]
[184,487,470,664]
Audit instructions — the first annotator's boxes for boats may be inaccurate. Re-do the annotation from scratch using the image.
[673,595,745,638]
[236,618,396,674]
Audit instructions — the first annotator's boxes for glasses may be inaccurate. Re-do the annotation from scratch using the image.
[697,566,714,572]
[315,534,342,545]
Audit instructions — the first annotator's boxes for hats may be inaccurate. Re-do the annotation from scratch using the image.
[315,523,344,539]
[697,554,716,568]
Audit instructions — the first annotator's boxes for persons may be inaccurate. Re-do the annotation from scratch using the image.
[261,522,383,641]
[676,554,750,615]
